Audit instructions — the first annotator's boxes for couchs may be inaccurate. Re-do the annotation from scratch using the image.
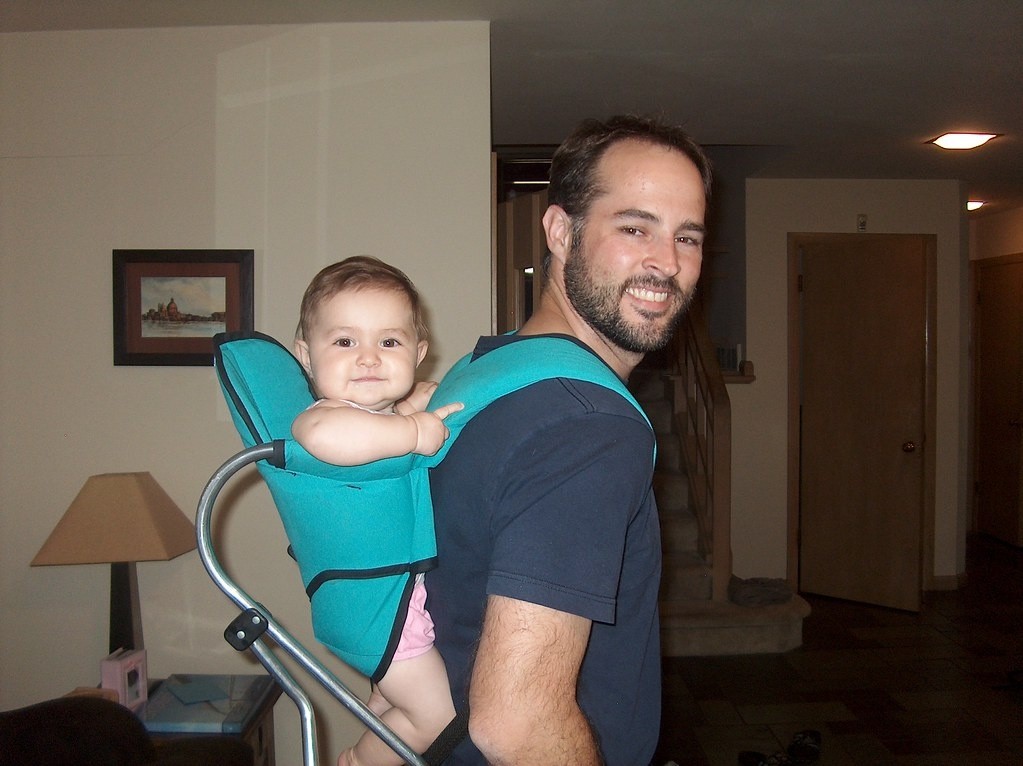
[0,699,256,766]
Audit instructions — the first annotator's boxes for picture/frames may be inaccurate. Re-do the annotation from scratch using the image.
[113,247,255,365]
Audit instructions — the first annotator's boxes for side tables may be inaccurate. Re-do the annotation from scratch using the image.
[61,674,284,766]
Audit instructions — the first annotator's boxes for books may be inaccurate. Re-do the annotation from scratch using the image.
[139,673,284,733]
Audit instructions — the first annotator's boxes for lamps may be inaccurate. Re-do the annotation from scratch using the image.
[28,471,198,656]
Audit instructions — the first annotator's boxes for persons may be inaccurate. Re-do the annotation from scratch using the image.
[423,113,715,766]
[290,255,466,766]
